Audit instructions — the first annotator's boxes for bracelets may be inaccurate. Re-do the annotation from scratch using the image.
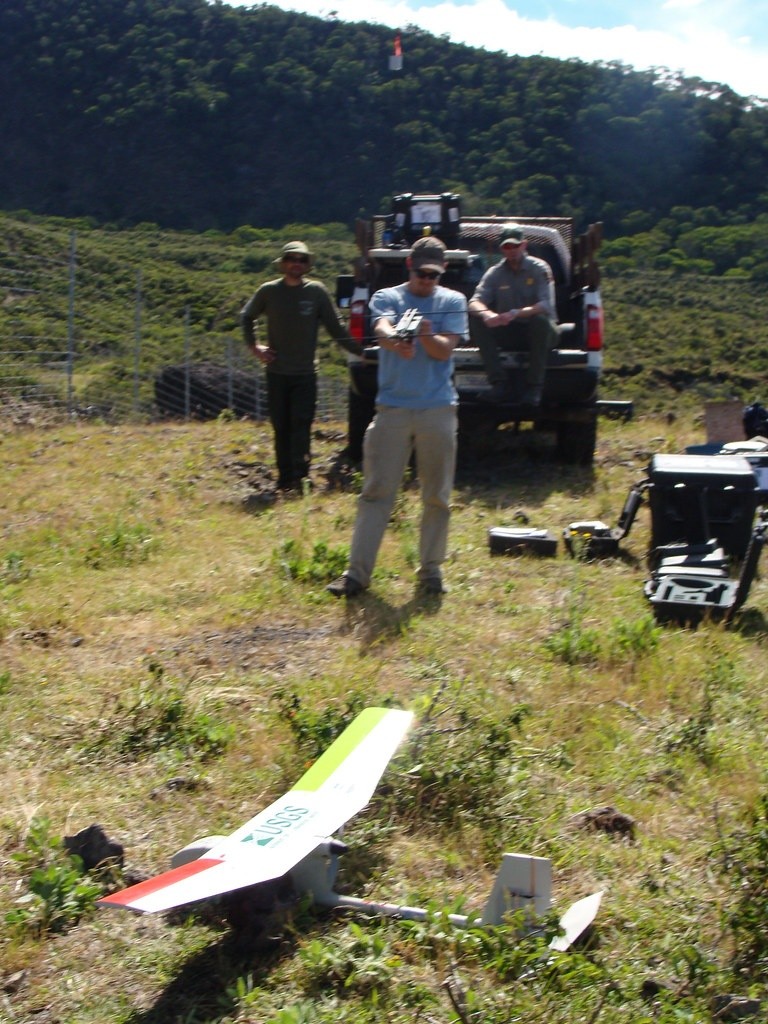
[510,308,519,318]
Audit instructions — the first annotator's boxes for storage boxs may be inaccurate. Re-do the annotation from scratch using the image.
[650,453,758,559]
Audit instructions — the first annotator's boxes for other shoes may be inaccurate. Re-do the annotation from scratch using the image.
[420,577,442,594]
[325,574,366,593]
[477,379,516,404]
[521,384,545,407]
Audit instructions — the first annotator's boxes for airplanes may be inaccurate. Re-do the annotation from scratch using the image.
[97,706,603,981]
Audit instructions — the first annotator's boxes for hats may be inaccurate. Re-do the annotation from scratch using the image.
[271,240,316,273]
[497,228,523,248]
[412,236,447,274]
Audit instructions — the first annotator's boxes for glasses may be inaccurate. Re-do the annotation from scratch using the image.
[283,255,308,264]
[414,269,441,280]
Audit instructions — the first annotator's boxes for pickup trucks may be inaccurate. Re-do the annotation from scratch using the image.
[335,193,603,470]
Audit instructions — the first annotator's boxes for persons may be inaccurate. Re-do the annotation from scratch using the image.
[241,239,365,490]
[328,236,470,594]
[466,220,559,405]
